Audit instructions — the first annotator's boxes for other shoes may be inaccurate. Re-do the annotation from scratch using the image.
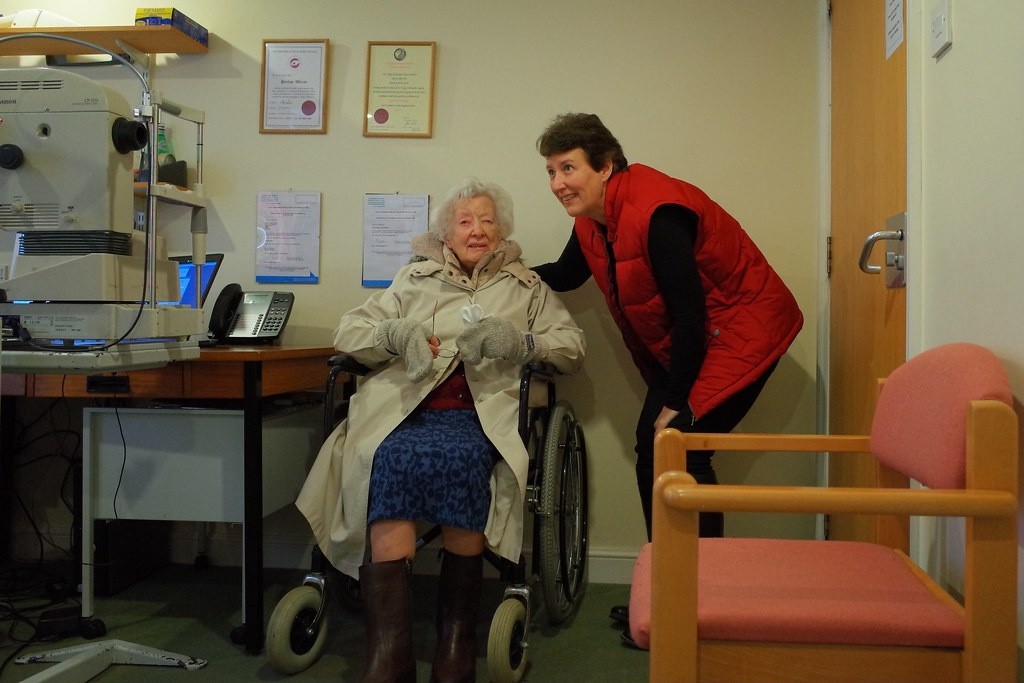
[608,605,631,621]
[622,630,636,643]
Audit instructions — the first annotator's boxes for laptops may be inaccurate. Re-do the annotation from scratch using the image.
[53,253,225,348]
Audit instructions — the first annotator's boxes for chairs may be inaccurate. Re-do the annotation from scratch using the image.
[629,342,1019,683]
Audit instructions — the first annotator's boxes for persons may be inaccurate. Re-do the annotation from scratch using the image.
[525,111,806,647]
[336,180,588,683]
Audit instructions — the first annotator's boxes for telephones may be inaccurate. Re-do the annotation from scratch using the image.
[206,282,296,345]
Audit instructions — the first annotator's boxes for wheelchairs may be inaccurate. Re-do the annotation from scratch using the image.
[265,350,589,683]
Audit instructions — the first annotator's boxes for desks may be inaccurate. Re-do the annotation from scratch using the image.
[0,345,356,657]
[77,400,327,644]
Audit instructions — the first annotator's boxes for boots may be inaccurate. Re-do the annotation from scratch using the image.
[357,556,417,683]
[431,549,486,683]
